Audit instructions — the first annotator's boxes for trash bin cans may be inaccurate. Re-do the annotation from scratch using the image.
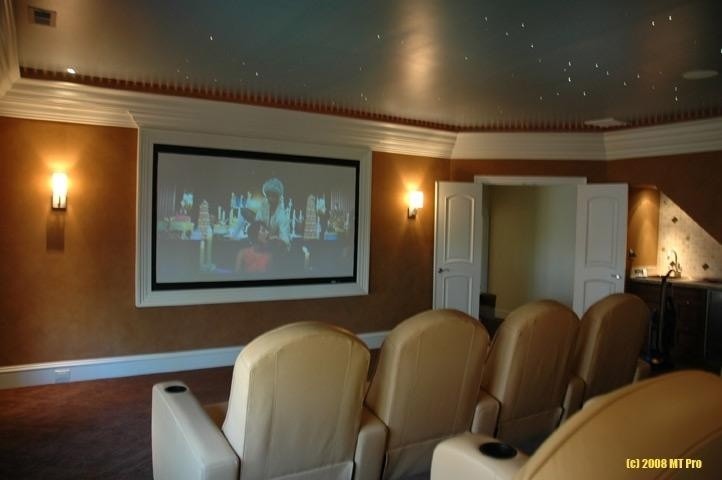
[479,293,496,321]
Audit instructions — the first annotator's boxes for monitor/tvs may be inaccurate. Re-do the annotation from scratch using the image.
[152,143,360,290]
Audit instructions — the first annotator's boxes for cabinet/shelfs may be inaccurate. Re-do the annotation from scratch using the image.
[629,278,721,362]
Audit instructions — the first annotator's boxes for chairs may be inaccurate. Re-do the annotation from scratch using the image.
[151,292,722,480]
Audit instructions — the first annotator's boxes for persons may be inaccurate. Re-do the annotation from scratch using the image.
[255,175,292,246]
[231,222,277,277]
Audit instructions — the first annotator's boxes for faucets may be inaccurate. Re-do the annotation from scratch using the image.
[667,250,677,269]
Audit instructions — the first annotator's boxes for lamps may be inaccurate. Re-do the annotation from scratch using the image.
[49,173,69,211]
[406,191,426,219]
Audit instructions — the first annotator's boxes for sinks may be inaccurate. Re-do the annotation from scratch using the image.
[648,275,665,279]
